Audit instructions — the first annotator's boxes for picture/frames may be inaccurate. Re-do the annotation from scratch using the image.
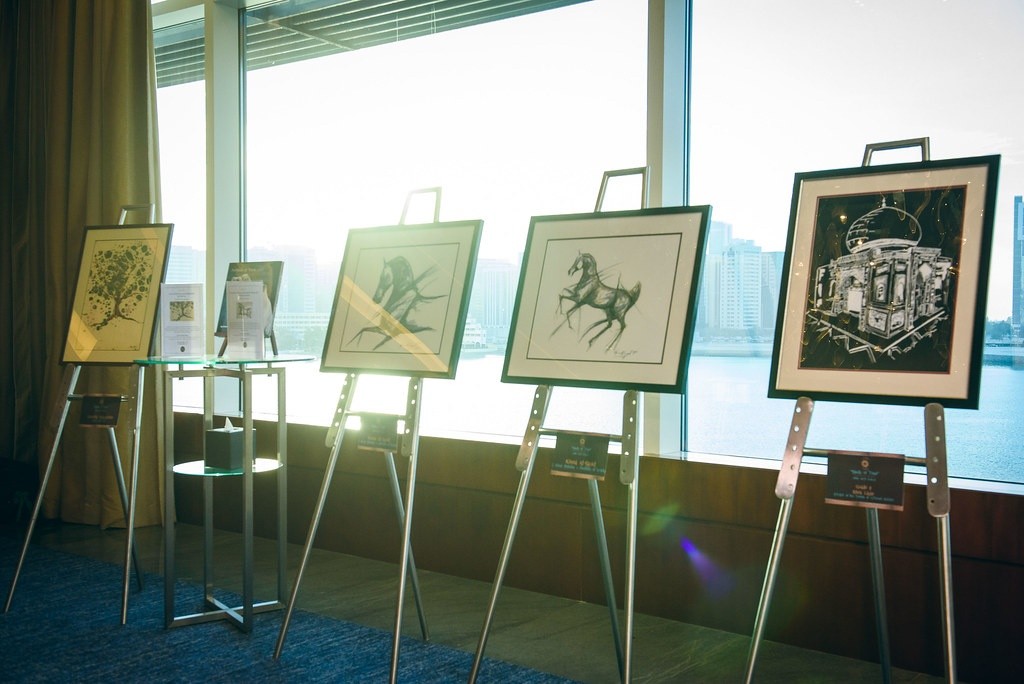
[319,219,484,378]
[59,223,174,366]
[767,154,1001,410]
[500,205,713,393]
[214,261,284,337]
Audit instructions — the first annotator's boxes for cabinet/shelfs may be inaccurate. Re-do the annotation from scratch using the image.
[132,356,315,633]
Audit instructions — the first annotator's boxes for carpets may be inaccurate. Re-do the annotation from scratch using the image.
[0,535,583,684]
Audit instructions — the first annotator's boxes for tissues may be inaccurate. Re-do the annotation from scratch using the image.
[203,416,257,471]
